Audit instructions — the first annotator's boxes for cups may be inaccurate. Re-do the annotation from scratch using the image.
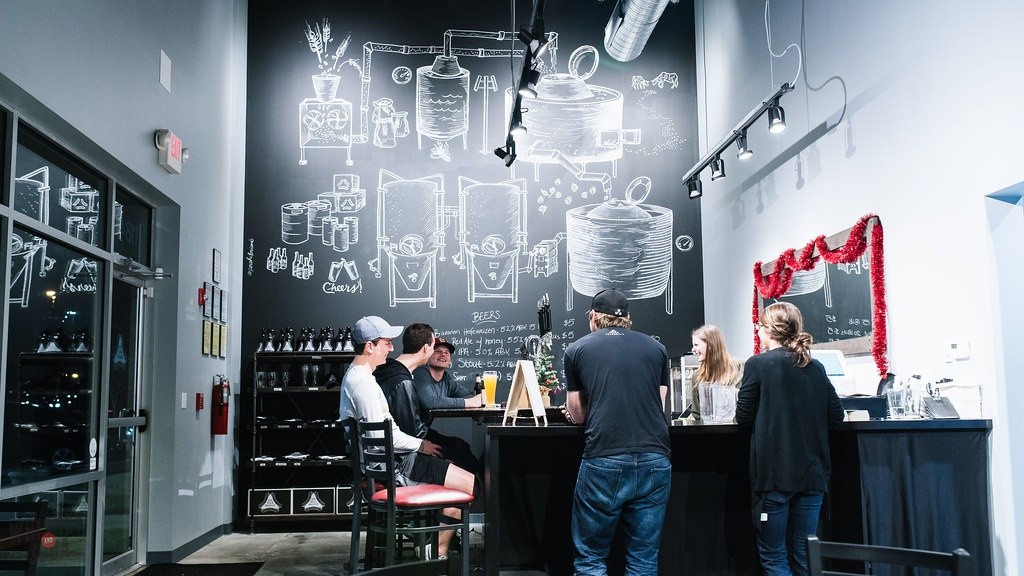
[483,371,497,408]
[257,364,320,388]
[886,388,906,419]
[905,385,922,419]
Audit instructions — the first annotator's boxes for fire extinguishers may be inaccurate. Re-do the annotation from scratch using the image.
[214,373,232,436]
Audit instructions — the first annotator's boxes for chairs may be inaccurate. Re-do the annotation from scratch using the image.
[348,416,475,575]
[807,534,972,576]
[337,417,445,574]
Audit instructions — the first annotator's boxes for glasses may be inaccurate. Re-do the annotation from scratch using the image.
[753,321,769,331]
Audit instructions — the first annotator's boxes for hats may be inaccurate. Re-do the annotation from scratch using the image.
[585,289,630,317]
[350,316,405,343]
[432,338,457,354]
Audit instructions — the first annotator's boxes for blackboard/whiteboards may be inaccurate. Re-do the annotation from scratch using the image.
[754,216,882,357]
[232,2,705,532]
[1,131,151,480]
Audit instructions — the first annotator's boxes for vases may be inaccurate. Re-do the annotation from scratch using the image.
[539,388,552,407]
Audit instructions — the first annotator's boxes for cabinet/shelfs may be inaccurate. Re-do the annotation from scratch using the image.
[247,350,376,534]
[3,351,94,535]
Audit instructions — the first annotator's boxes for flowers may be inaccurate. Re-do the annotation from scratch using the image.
[532,354,562,396]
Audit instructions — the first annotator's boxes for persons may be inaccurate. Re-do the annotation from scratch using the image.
[689,323,746,423]
[372,322,467,552]
[339,313,481,566]
[410,337,484,482]
[735,303,844,576]
[560,288,672,576]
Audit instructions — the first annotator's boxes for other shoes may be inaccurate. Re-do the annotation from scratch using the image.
[448,535,463,555]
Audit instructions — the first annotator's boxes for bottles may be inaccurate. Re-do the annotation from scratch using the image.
[472,374,484,407]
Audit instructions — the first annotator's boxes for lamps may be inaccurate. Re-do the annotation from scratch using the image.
[515,18,549,60]
[493,148,516,168]
[735,127,753,160]
[517,62,541,100]
[182,148,190,163]
[768,97,786,135]
[687,175,703,200]
[509,107,529,137]
[710,154,726,182]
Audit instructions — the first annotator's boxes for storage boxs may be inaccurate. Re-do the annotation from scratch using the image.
[246,482,373,517]
[14,491,89,520]
[8,469,48,486]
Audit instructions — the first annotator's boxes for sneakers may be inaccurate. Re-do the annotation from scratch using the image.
[413,543,449,563]
